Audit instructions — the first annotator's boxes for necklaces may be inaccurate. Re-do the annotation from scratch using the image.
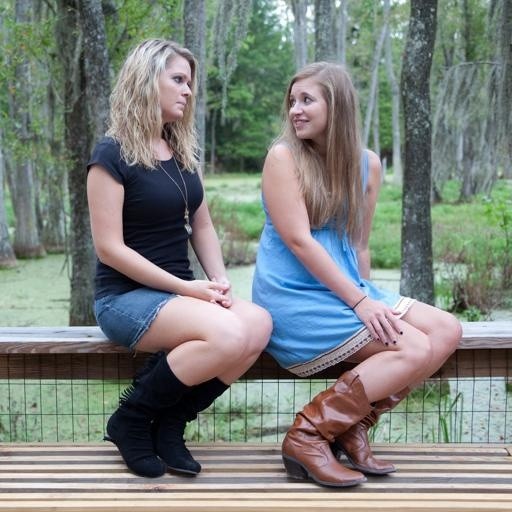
[152,151,193,237]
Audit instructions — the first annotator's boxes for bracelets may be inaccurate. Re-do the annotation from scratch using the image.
[351,295,368,311]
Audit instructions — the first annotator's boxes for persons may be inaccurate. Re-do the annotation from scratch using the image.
[84,38,276,478]
[251,59,463,488]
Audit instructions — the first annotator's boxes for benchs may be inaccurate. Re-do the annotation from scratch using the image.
[1,323,512,385]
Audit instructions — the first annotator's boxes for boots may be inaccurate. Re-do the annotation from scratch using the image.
[281,372,411,487]
[106,350,231,478]
